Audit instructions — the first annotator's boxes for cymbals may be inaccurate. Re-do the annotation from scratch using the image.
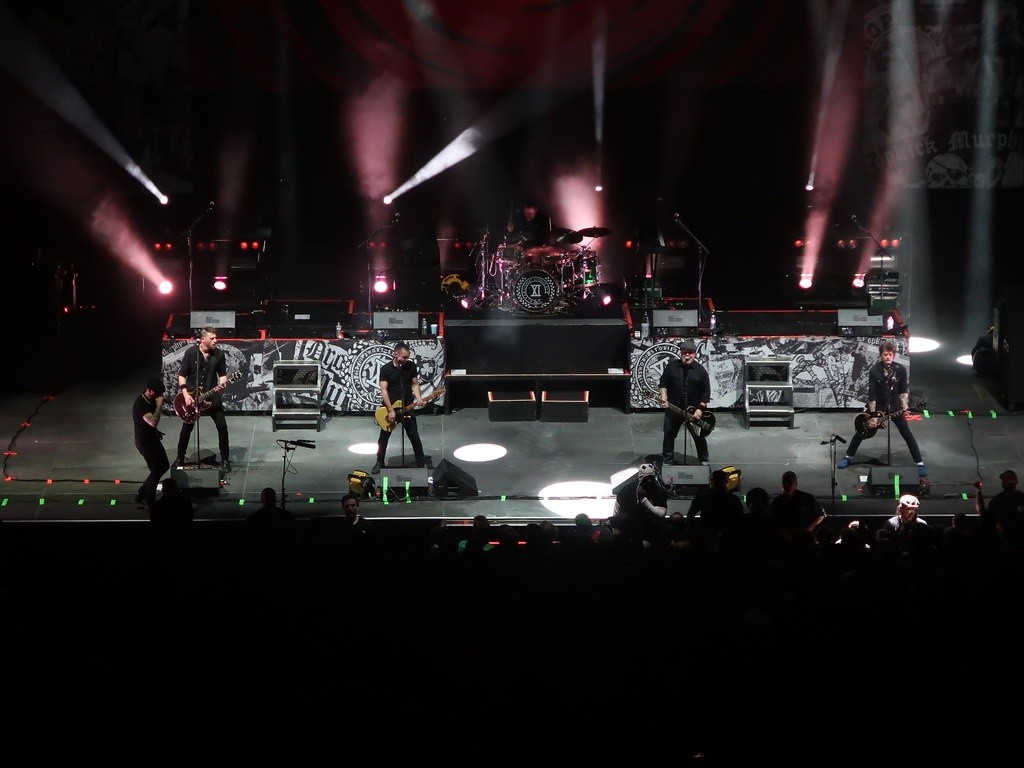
[579,227,613,237]
[553,228,583,244]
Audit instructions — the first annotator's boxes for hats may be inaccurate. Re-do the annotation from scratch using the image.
[680,341,696,353]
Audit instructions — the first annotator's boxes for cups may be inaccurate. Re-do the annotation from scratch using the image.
[430,324,438,336]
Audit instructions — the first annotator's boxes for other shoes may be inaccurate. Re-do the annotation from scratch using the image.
[418,459,433,469]
[919,466,928,476]
[701,460,711,466]
[221,458,231,473]
[838,458,851,469]
[372,462,385,474]
[138,487,155,501]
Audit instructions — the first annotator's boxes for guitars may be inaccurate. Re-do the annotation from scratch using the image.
[854,400,928,439]
[173,369,244,424]
[640,388,717,438]
[374,386,447,432]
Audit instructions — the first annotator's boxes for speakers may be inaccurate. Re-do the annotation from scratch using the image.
[432,458,478,501]
[372,311,419,340]
[653,309,698,339]
[867,466,920,497]
[837,309,884,337]
[378,468,429,500]
[610,456,646,494]
[171,467,219,496]
[661,465,710,496]
[190,310,236,339]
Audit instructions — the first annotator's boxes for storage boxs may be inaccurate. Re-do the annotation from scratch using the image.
[542,390,590,423]
[488,389,536,422]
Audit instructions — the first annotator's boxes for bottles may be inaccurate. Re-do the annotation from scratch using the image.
[643,311,651,335]
[421,318,427,335]
[887,316,894,331]
[336,322,342,339]
[710,315,716,336]
[641,321,649,340]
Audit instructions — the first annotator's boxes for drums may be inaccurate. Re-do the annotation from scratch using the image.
[496,244,525,266]
[561,250,597,281]
[514,268,558,313]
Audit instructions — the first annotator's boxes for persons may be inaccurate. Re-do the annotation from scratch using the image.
[658,341,710,465]
[370,342,435,475]
[132,379,170,510]
[506,202,551,245]
[837,342,928,478]
[169,326,231,474]
[0,470,1024,768]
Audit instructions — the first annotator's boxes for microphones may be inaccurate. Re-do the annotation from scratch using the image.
[685,357,690,365]
[209,201,215,210]
[886,361,891,368]
[196,339,200,346]
[398,357,403,366]
[395,213,400,222]
[277,439,316,449]
[675,213,679,222]
[852,215,857,223]
[832,435,847,445]
